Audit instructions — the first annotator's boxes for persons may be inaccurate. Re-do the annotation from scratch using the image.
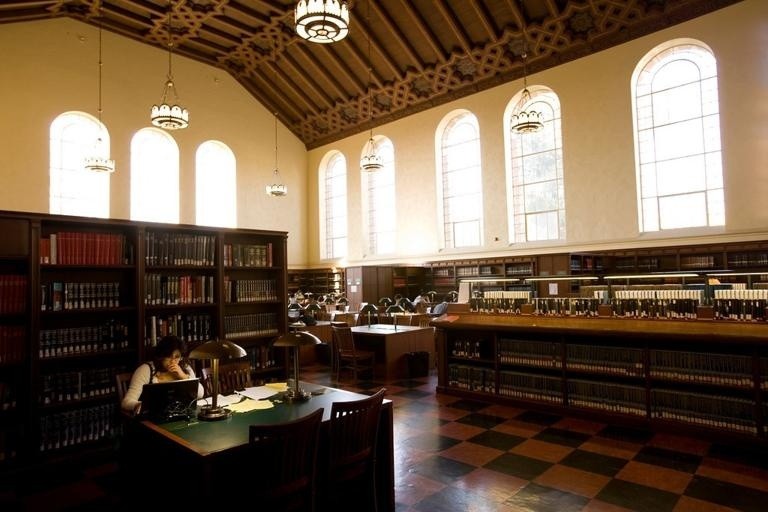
[121,334,206,416]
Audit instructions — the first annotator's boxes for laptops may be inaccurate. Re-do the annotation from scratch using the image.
[137,378,198,421]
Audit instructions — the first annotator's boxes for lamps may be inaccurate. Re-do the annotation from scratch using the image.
[361,1,385,174]
[266,68,288,197]
[509,2,544,135]
[294,0,350,44]
[189,334,246,423]
[84,6,119,176]
[151,0,191,132]
[270,330,323,404]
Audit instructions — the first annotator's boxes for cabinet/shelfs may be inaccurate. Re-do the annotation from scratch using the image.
[1,208,28,479]
[140,221,225,356]
[28,212,140,469]
[424,236,768,307]
[223,230,292,382]
[286,268,346,292]
[346,263,391,310]
[391,265,424,298]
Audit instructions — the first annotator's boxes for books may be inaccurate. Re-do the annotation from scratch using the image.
[39,324,129,405]
[41,404,117,453]
[393,267,432,301]
[39,231,133,310]
[506,264,532,291]
[224,312,283,386]
[614,257,715,271]
[290,273,347,296]
[143,231,216,305]
[470,282,768,323]
[726,251,768,269]
[223,243,281,302]
[433,268,456,294]
[145,314,213,377]
[570,256,607,288]
[456,266,495,277]
[444,331,768,439]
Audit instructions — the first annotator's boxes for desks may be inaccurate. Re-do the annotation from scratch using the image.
[127,382,394,510]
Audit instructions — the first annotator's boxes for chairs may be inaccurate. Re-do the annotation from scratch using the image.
[115,367,135,401]
[202,357,251,399]
[285,290,458,389]
[316,385,390,512]
[231,407,329,512]
